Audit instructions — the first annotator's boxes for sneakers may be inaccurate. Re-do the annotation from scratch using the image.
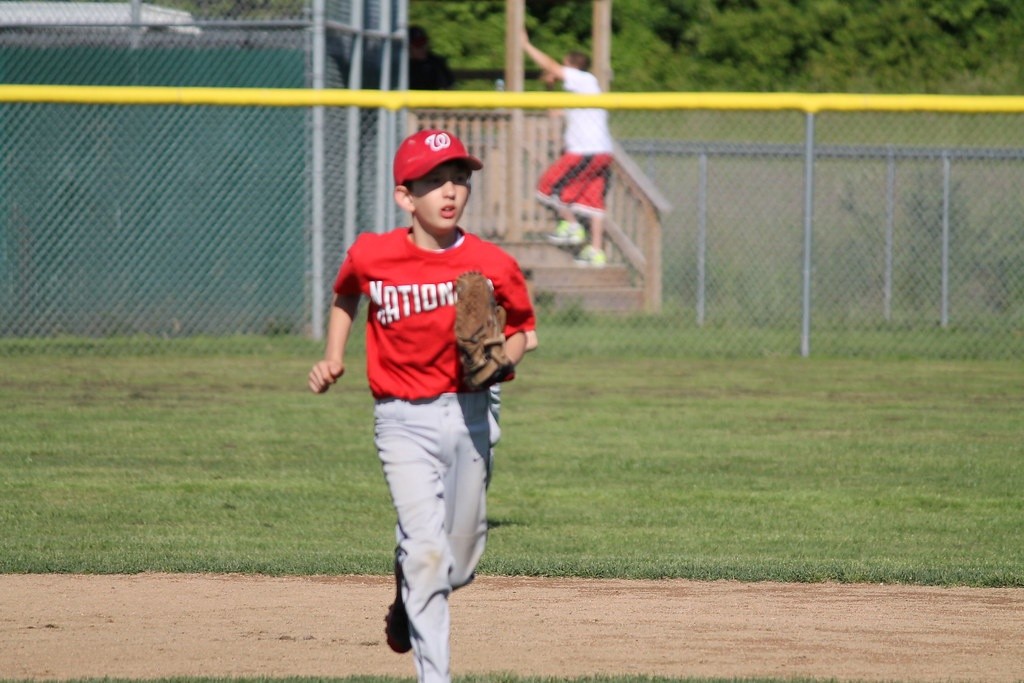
[569,245,606,270]
[543,220,585,244]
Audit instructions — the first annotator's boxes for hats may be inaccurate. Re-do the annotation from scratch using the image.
[393,129,484,186]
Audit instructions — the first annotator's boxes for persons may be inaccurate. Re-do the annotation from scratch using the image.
[523,28,613,268]
[309,129,539,683]
[392,25,455,91]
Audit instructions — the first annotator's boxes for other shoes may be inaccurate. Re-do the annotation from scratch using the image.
[385,546,412,653]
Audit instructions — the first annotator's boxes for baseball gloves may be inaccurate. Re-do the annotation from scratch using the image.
[454,271,514,390]
